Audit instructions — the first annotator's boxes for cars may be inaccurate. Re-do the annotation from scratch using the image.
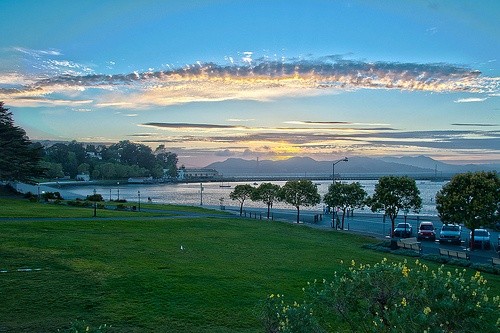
[394,223,412,238]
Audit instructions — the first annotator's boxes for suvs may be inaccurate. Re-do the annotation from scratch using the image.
[417,222,436,242]
[439,224,462,245]
[469,229,490,250]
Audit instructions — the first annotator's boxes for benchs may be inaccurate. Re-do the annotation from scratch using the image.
[400,237,417,242]
[441,249,472,260]
[397,242,423,252]
[491,257,500,266]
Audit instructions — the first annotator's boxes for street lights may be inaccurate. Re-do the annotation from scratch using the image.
[402,210,408,239]
[92,188,97,217]
[333,157,349,229]
